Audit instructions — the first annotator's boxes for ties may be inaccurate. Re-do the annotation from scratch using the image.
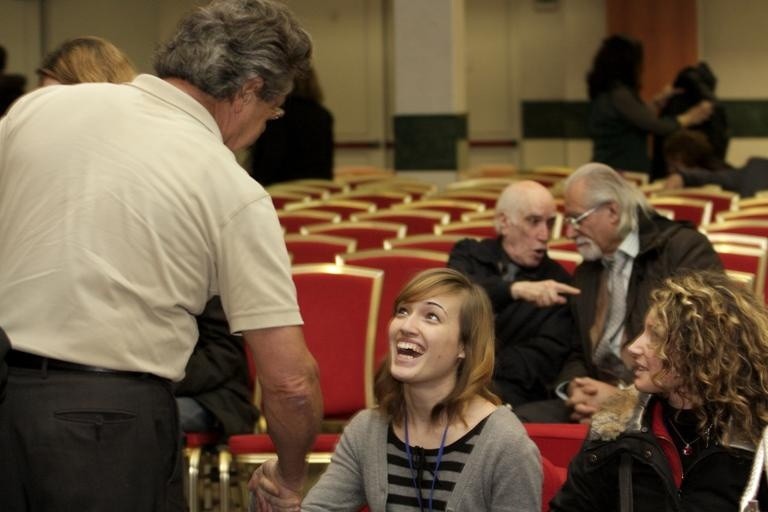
[501,262,519,283]
[591,251,633,384]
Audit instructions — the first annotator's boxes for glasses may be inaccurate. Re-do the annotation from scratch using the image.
[564,209,598,227]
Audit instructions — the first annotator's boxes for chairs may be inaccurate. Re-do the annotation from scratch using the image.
[178,160,768,510]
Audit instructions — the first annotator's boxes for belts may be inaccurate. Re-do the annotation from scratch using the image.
[4,349,172,384]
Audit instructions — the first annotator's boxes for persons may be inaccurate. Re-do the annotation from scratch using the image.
[512,162,728,423]
[547,267,768,512]
[585,31,715,171]
[649,129,739,191]
[657,61,729,159]
[447,179,581,408]
[247,268,545,512]
